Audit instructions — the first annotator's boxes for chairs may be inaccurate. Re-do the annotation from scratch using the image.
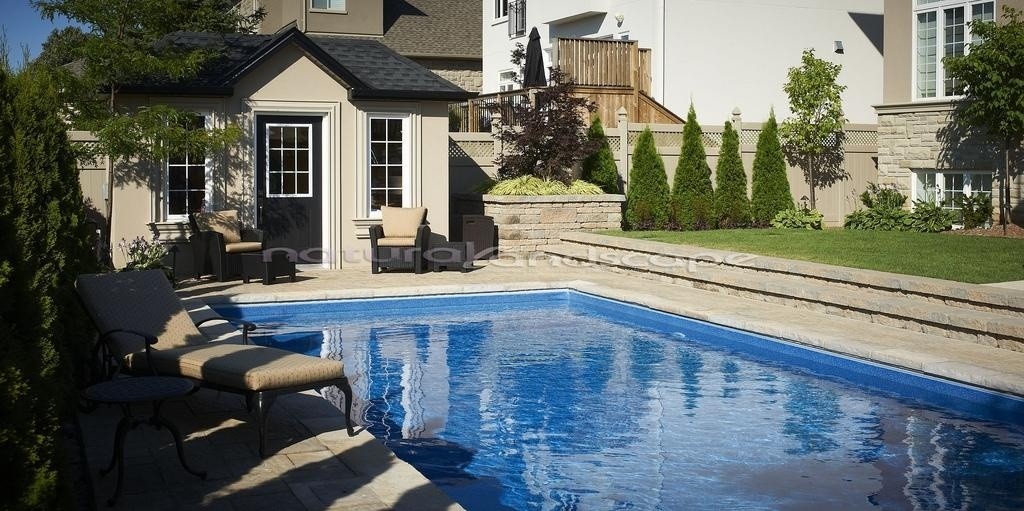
[185,205,264,283]
[70,263,357,462]
[368,203,428,273]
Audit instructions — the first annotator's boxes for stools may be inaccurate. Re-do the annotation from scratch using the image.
[430,240,475,274]
[242,250,296,285]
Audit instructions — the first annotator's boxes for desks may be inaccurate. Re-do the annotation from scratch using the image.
[83,368,215,507]
